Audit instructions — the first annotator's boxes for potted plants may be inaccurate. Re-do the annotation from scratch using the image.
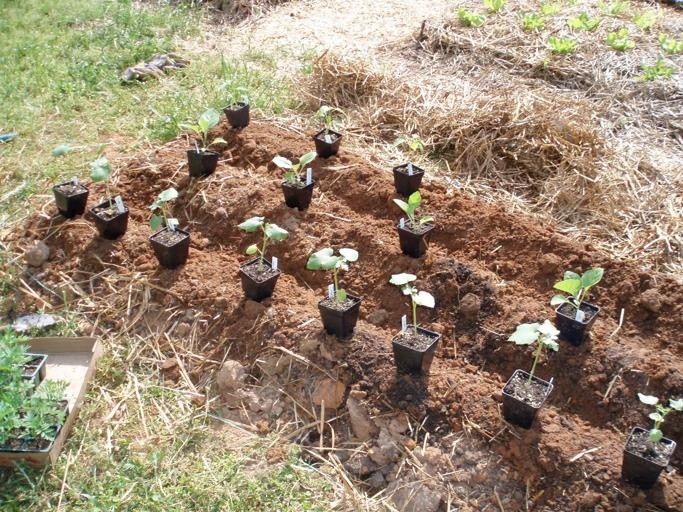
[237,216,290,303]
[549,267,606,343]
[389,273,442,374]
[54,143,129,239]
[220,83,250,128]
[620,393,682,489]
[499,319,560,430]
[1,323,74,455]
[393,137,426,198]
[312,105,348,158]
[307,247,363,338]
[272,152,320,209]
[392,192,436,257]
[179,107,228,177]
[148,187,191,269]
